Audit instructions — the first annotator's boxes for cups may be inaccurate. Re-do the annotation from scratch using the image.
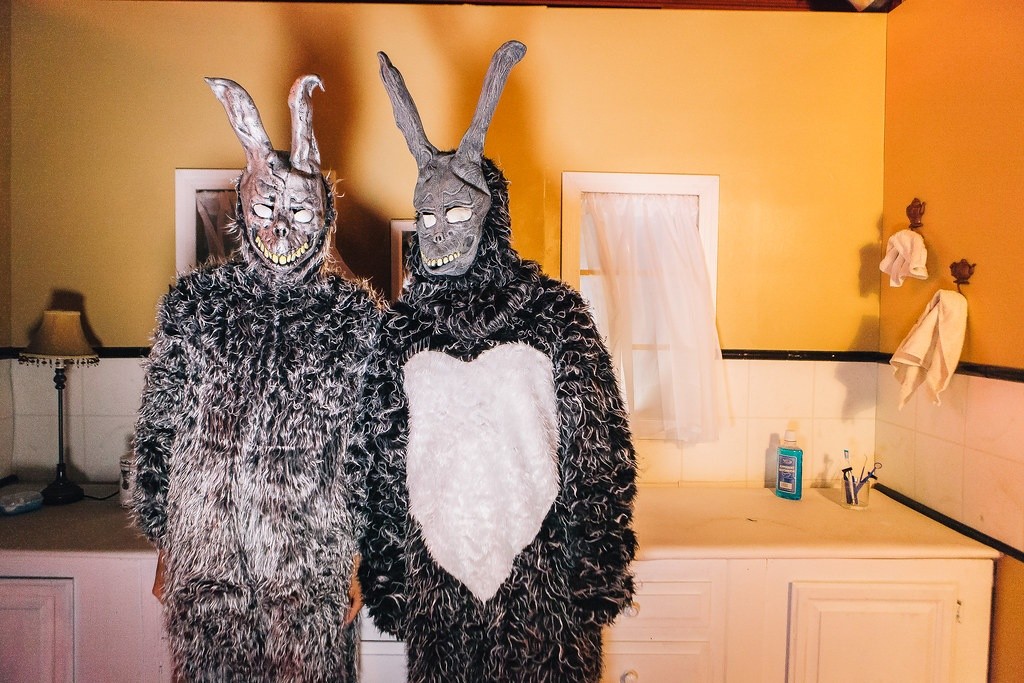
[119,454,136,509]
[843,478,870,511]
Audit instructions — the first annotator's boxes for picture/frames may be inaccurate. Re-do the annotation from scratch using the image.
[174,167,336,280]
[391,216,421,305]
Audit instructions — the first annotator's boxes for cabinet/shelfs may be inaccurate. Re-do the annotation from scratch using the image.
[1,483,1003,683]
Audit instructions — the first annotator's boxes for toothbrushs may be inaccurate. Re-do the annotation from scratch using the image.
[842,449,858,506]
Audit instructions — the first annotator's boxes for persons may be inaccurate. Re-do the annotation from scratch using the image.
[120,66,392,680]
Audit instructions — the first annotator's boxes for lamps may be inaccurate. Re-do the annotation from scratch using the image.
[17,310,100,505]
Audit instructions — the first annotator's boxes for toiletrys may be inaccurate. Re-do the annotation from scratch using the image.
[775,429,804,501]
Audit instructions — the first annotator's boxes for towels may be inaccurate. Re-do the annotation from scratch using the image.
[880,228,969,411]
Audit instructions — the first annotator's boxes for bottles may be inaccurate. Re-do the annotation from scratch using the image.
[775,430,803,500]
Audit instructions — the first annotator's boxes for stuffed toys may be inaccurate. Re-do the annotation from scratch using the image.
[342,35,645,683]
